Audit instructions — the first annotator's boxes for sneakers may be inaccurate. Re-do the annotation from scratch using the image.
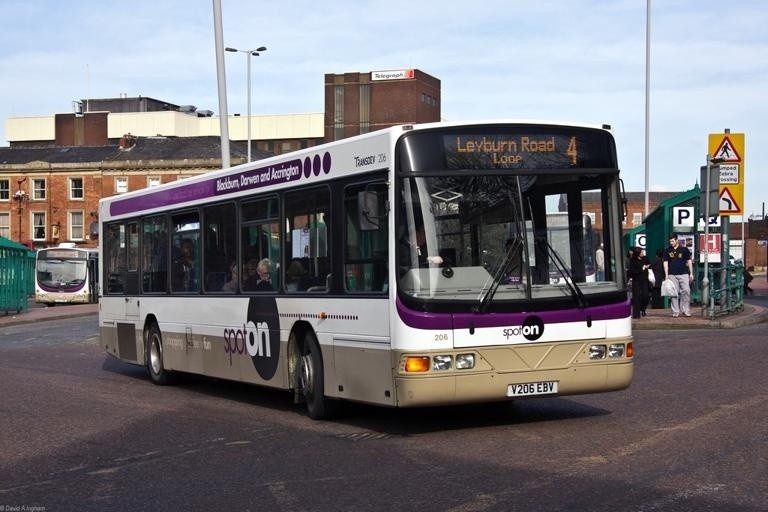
[672,313,679,317]
[682,311,692,317]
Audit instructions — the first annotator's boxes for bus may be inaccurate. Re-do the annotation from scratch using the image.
[95,115,638,415]
[0,249,35,297]
[516,206,608,287]
[165,221,218,253]
[35,241,101,308]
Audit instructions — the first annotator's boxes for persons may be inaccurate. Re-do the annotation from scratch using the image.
[595,241,608,282]
[149,214,329,297]
[506,239,528,283]
[626,246,657,315]
[658,233,694,317]
[624,245,636,301]
[391,218,436,286]
[744,271,754,294]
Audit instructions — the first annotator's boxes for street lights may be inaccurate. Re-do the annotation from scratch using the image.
[225,42,269,165]
[12,190,29,243]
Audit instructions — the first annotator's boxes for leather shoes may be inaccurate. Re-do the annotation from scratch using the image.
[641,308,646,316]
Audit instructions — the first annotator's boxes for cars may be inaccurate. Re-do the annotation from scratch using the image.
[729,255,754,294]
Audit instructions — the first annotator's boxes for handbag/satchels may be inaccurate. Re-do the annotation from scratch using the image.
[647,267,655,286]
[660,280,678,296]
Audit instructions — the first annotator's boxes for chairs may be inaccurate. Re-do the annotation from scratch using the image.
[438,248,456,267]
